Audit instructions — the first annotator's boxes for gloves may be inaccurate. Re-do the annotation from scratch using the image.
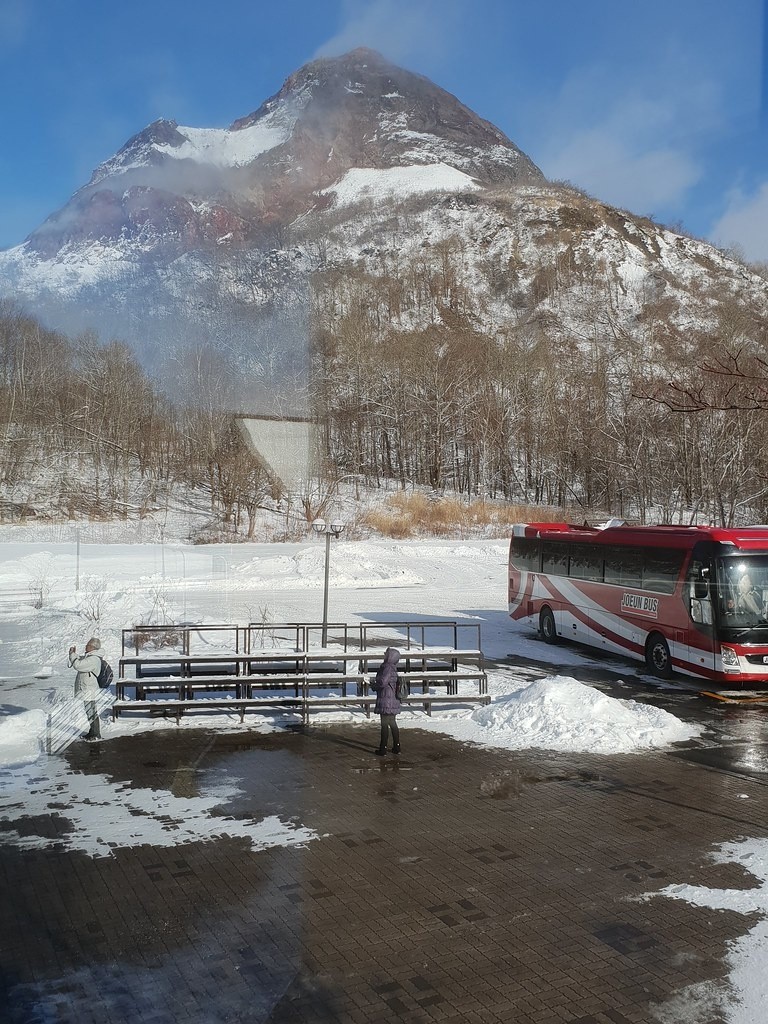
[369,681,376,691]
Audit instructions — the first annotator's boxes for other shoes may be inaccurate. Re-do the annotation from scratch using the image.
[80,733,102,742]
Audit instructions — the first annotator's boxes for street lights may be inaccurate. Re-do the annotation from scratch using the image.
[312,518,346,648]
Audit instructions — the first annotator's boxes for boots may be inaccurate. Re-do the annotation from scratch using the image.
[375,744,388,756]
[392,742,401,754]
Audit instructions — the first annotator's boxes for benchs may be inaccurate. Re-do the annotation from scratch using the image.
[112,621,492,726]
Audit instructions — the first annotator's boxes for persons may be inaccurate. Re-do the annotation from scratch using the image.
[69,637,105,742]
[371,646,401,755]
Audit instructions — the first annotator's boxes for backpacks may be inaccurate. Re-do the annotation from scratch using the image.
[86,655,113,688]
[387,663,408,704]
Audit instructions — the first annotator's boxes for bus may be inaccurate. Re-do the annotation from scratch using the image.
[509,523,768,683]
